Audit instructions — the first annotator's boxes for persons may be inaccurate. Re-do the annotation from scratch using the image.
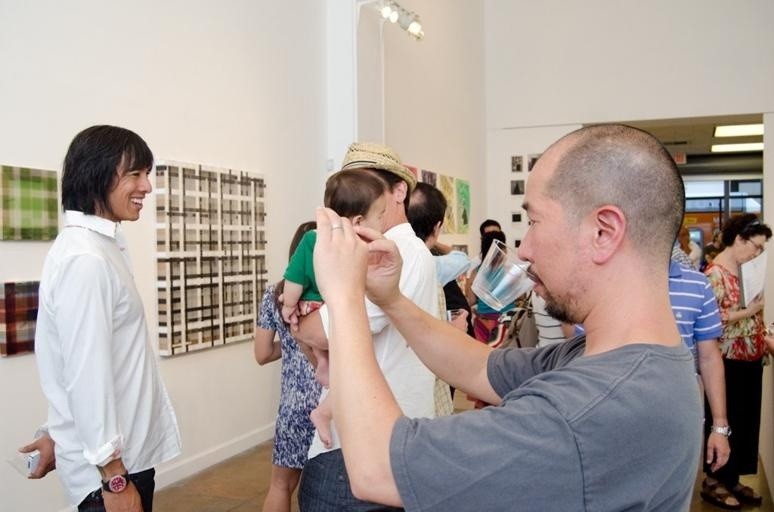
[251,221,322,511]
[13,121,183,511]
[676,226,700,270]
[702,211,774,511]
[404,181,577,411]
[278,166,388,448]
[308,123,706,512]
[704,229,725,264]
[287,139,439,509]
[572,259,734,477]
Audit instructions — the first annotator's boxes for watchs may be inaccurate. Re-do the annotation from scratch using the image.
[710,423,732,438]
[99,471,131,492]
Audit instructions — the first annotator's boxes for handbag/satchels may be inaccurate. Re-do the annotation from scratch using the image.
[498,311,538,347]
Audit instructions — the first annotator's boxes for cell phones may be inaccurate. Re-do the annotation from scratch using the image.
[27,449,41,475]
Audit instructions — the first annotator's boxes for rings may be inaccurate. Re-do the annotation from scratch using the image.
[331,224,343,229]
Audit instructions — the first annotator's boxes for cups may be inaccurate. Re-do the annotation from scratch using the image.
[471,238,540,312]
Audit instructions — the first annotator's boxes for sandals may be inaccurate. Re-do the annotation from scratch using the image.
[730,482,763,503]
[700,478,742,510]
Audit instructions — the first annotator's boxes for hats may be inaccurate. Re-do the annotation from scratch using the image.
[341,142,417,192]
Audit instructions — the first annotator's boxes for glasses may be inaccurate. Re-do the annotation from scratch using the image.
[748,239,764,254]
